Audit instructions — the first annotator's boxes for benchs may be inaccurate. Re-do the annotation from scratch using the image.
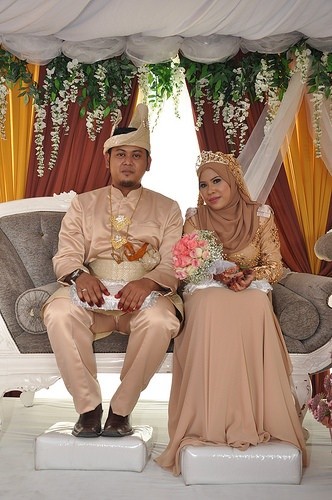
[0,190,332,447]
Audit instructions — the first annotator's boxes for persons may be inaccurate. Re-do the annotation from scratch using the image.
[39,116,186,438]
[154,150,311,477]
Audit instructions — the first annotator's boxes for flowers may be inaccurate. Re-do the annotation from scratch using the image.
[172,230,248,291]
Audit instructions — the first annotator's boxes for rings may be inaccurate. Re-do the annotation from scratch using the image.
[81,288,87,292]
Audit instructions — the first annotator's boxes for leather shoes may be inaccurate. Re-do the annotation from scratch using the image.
[101,404,134,437]
[72,404,104,437]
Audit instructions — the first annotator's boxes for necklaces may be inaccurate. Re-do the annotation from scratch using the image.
[108,185,143,264]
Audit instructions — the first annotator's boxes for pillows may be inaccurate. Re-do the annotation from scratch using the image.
[15,281,60,334]
[267,280,320,340]
